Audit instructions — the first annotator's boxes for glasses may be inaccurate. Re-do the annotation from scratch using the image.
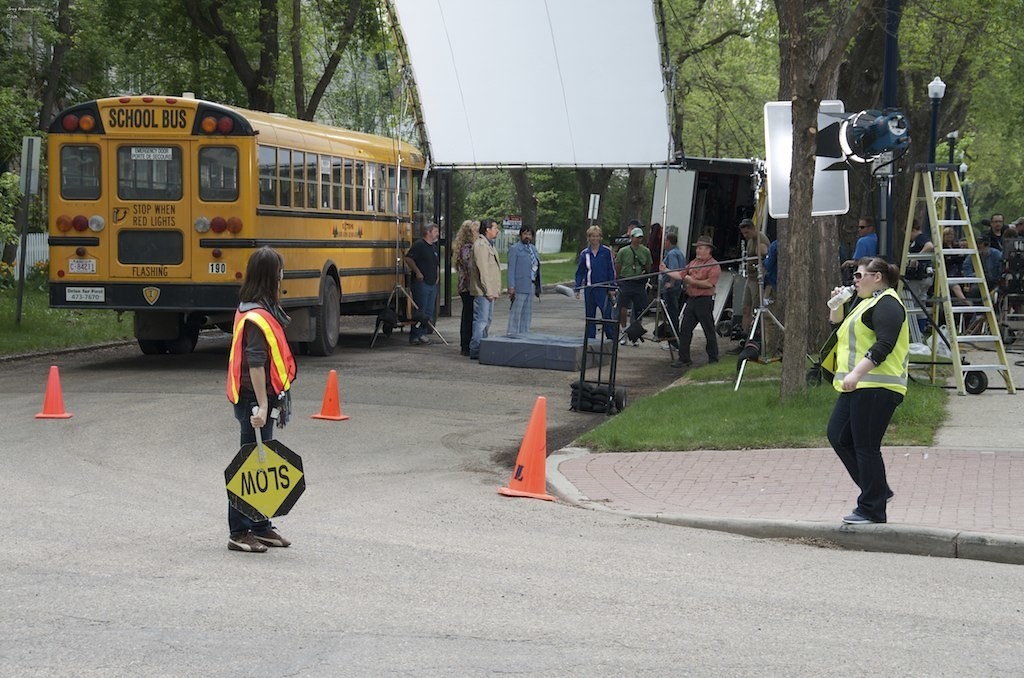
[853,271,877,281]
[858,225,869,229]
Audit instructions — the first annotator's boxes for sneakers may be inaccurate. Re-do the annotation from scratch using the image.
[633,342,638,346]
[409,337,422,344]
[227,531,268,552]
[420,335,432,344]
[251,529,291,547]
[620,337,626,344]
[662,344,679,351]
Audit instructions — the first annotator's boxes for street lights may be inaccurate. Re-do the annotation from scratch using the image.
[925,77,970,262]
[34,364,71,420]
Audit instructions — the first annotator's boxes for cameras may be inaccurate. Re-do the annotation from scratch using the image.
[639,265,648,284]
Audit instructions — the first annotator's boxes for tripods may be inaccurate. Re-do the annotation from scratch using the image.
[618,91,679,356]
[366,93,447,351]
[814,166,953,374]
[732,197,820,391]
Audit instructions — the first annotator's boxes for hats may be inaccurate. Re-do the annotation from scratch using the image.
[691,236,716,249]
[976,236,991,244]
[630,227,644,238]
[627,219,645,227]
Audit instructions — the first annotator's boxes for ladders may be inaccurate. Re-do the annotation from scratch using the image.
[894,162,1016,395]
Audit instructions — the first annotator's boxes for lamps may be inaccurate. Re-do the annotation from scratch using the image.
[817,109,911,263]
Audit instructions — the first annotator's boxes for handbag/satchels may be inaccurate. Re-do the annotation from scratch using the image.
[611,305,619,320]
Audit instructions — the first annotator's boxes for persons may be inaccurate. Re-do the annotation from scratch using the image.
[724,218,773,357]
[825,258,911,526]
[223,246,299,553]
[839,216,880,314]
[612,220,646,267]
[613,227,654,347]
[898,218,935,338]
[940,210,1024,342]
[506,225,544,338]
[405,221,442,345]
[646,222,664,292]
[468,218,503,360]
[659,232,686,352]
[659,235,722,369]
[573,226,617,341]
[761,239,779,364]
[452,220,481,357]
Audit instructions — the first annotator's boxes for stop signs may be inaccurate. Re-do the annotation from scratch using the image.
[225,439,305,524]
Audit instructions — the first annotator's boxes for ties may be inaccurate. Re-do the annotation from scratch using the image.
[527,246,535,267]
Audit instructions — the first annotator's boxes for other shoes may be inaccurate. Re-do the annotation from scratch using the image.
[671,360,693,368]
[461,350,470,356]
[470,351,480,358]
[842,513,875,524]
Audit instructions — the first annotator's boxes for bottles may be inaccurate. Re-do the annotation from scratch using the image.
[827,284,856,311]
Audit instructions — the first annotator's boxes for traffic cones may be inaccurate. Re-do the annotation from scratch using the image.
[496,396,557,504]
[311,368,347,424]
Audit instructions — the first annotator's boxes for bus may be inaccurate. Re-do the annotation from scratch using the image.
[45,99,427,356]
